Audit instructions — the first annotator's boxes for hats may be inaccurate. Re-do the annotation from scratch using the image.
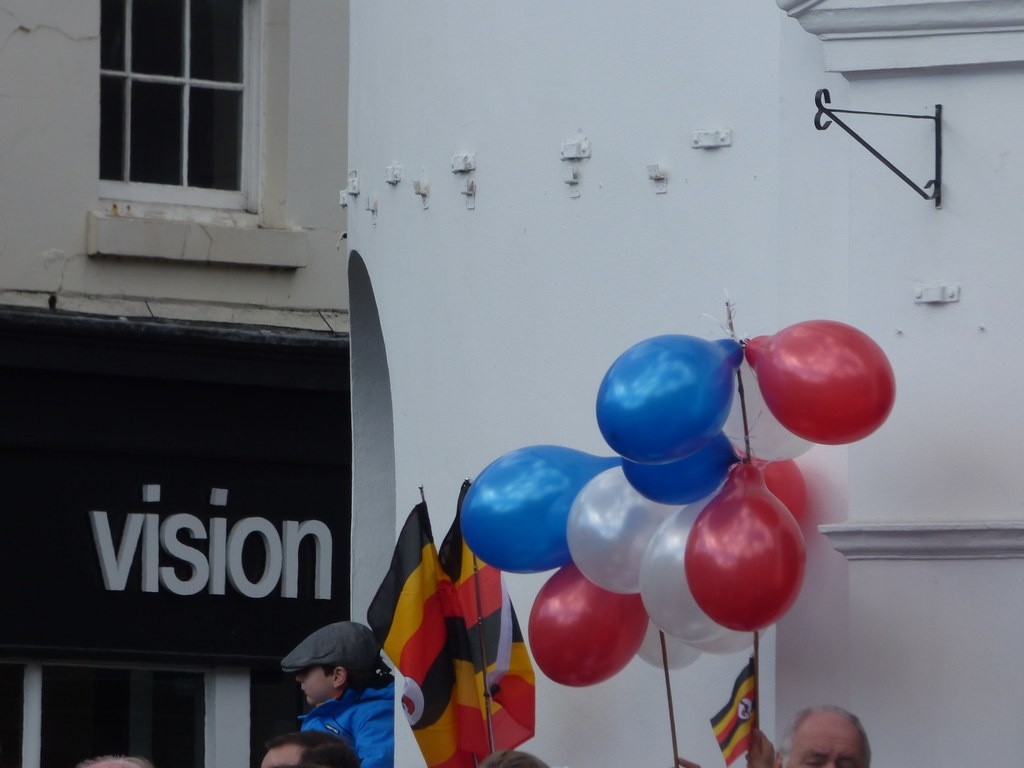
[281,623,391,675]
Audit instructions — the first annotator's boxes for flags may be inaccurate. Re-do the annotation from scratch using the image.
[438,481,536,768]
[709,657,756,768]
[367,501,490,768]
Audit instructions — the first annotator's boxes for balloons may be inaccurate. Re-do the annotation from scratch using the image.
[636,336,816,671]
[622,430,742,506]
[684,458,807,632]
[745,319,897,445]
[528,563,649,688]
[460,445,622,574]
[595,334,744,465]
[567,466,688,594]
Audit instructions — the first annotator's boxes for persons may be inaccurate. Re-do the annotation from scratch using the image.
[261,731,359,768]
[281,621,395,768]
[774,705,871,768]
[744,728,775,768]
